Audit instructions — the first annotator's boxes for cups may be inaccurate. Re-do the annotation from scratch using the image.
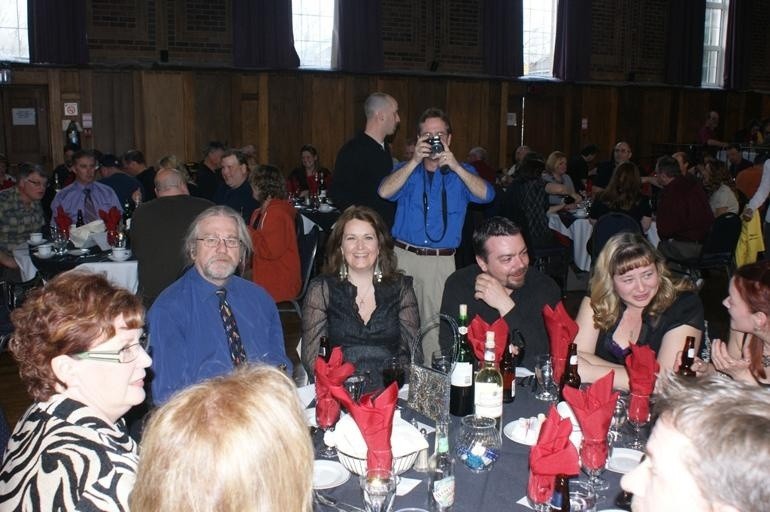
[575,208,586,216]
[27,227,130,262]
[567,479,598,512]
[358,469,398,512]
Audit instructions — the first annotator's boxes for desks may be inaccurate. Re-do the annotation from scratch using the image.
[716,150,755,168]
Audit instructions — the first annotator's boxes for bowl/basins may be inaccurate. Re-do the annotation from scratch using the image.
[323,430,428,477]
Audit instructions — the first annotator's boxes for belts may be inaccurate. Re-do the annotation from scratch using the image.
[394,240,454,256]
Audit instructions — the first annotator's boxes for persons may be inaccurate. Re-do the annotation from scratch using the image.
[439,215,560,372]
[377,108,495,368]
[0,274,153,512]
[573,232,705,393]
[146,206,292,404]
[129,363,314,512]
[693,263,769,388]
[301,206,423,393]
[1,90,769,344]
[620,363,770,512]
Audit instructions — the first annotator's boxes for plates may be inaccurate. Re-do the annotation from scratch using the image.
[572,213,588,219]
[312,458,351,490]
[604,447,645,474]
[503,418,546,446]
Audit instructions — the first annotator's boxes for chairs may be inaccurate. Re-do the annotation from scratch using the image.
[275,213,319,320]
[735,164,763,199]
[665,212,741,296]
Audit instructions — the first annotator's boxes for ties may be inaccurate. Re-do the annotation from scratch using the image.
[215,288,248,371]
[83,188,98,224]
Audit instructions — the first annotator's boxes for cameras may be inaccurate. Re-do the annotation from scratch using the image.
[423,135,444,154]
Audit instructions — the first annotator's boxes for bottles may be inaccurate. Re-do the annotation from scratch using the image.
[473,331,504,434]
[454,415,504,473]
[449,305,475,418]
[53,172,63,193]
[75,208,85,228]
[549,473,572,512]
[133,194,144,211]
[500,333,516,403]
[429,415,454,512]
[586,179,594,198]
[65,121,80,147]
[559,342,581,403]
[678,335,697,379]
[317,336,329,361]
[122,199,131,229]
[110,206,119,226]
[286,172,336,212]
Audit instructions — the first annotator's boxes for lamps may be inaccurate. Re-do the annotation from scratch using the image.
[429,57,439,72]
[160,50,168,62]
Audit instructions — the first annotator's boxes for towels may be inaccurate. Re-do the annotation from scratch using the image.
[543,301,578,382]
[624,341,660,421]
[735,208,766,269]
[329,380,398,480]
[325,175,333,192]
[307,176,319,195]
[525,404,581,505]
[286,177,299,196]
[467,315,510,371]
[53,204,73,241]
[314,345,356,433]
[98,206,122,247]
[586,179,593,194]
[560,369,620,469]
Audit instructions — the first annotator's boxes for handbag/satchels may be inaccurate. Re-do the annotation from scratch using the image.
[407,316,461,424]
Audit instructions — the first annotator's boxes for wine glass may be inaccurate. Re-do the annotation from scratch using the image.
[383,356,405,390]
[610,390,627,440]
[579,433,610,490]
[626,392,651,449]
[526,470,550,512]
[534,353,559,402]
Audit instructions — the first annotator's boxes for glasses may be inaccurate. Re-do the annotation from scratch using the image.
[28,178,51,188]
[196,236,242,248]
[73,332,153,364]
